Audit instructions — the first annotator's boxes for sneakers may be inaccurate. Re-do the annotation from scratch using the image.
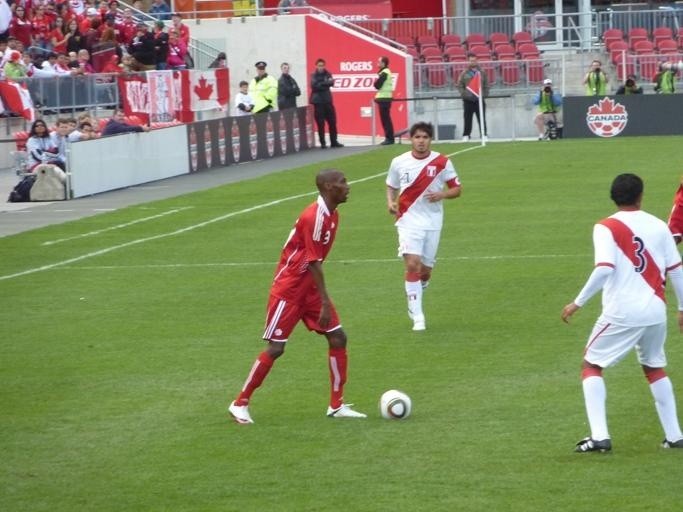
[661,439,682,449]
[413,317,425,330]
[228,400,253,423]
[575,437,612,452]
[327,404,366,418]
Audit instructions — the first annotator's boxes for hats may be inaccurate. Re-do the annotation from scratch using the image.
[255,62,267,67]
[544,79,553,84]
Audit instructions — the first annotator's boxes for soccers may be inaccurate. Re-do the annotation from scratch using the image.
[379,389,411,418]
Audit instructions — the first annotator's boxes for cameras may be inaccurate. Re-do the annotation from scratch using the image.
[544,86,550,93]
[625,78,635,87]
[595,68,601,74]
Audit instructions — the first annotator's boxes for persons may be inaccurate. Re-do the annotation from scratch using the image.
[27,110,150,174]
[247,61,277,114]
[226,167,370,426]
[580,59,610,96]
[383,120,462,331]
[232,79,254,117]
[530,77,565,141]
[651,61,678,94]
[559,172,682,454]
[613,75,645,95]
[308,58,344,149]
[372,54,395,145]
[666,180,683,248]
[276,61,301,109]
[456,52,489,142]
[276,0,312,17]
[209,52,228,68]
[0,0,191,118]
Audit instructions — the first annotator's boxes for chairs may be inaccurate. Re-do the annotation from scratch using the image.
[602,27,682,79]
[390,32,543,89]
[11,116,184,175]
